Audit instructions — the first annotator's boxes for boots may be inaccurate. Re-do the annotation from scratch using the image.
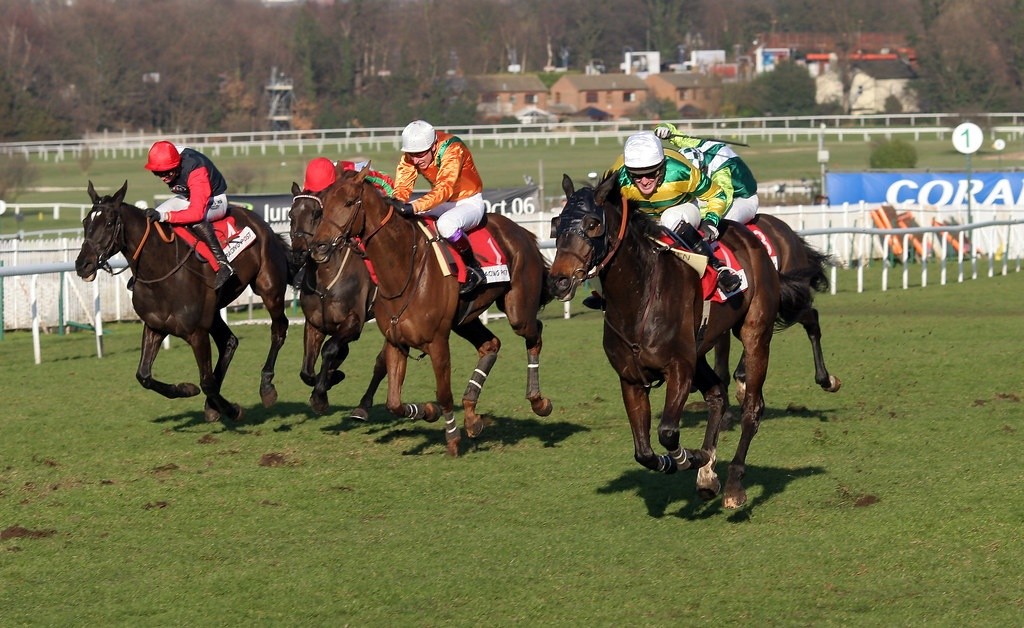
[451,232,486,293]
[582,294,607,310]
[194,221,232,290]
[676,222,741,293]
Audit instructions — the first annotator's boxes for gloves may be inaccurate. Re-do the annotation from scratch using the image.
[143,208,160,223]
[401,204,413,215]
[654,126,670,139]
[700,219,719,241]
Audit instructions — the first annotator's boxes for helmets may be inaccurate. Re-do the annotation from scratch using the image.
[145,142,180,171]
[304,158,335,193]
[401,120,436,153]
[624,134,664,174]
[678,147,704,169]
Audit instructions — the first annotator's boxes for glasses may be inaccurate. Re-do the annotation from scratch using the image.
[151,169,175,177]
[408,144,434,158]
[632,166,661,179]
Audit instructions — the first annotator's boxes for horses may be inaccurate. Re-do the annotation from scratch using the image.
[309,160,555,457]
[546,169,841,510]
[74,180,294,423]
[287,181,388,423]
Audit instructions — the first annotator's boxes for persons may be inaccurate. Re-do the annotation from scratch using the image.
[580,131,742,310]
[303,157,394,198]
[144,141,235,290]
[387,120,486,295]
[653,123,759,224]
